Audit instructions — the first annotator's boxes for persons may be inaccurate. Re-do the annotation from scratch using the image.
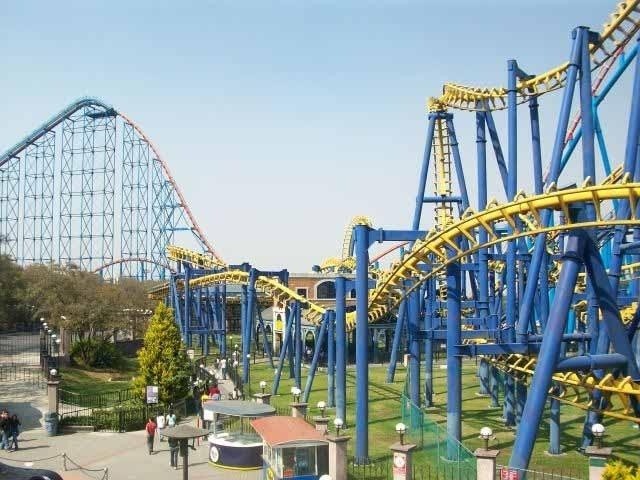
[156,410,166,442]
[1,409,23,453]
[165,410,176,427]
[145,416,156,455]
[192,382,247,414]
[168,436,178,470]
[219,357,228,381]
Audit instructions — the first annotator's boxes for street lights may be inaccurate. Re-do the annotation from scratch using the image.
[40,317,61,381]
[229,335,251,384]
